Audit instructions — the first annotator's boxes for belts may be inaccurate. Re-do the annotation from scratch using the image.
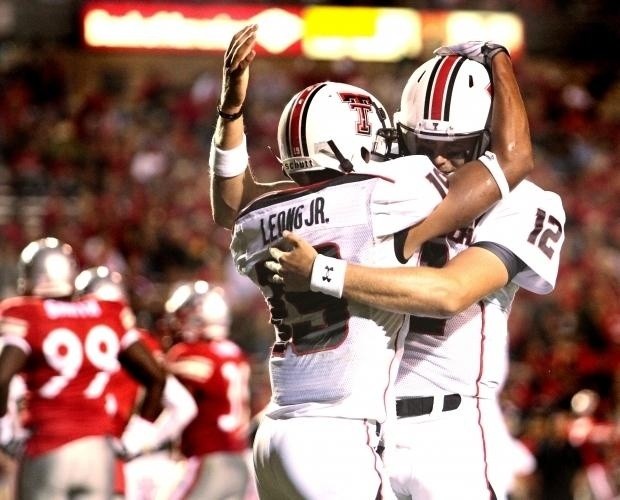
[396,393,462,418]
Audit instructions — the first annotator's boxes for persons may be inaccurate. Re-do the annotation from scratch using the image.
[73,266,199,500]
[207,26,567,500]
[0,237,166,500]
[231,41,536,500]
[160,280,250,500]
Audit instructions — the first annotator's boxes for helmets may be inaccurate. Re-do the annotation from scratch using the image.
[16,237,80,301]
[163,281,230,341]
[74,266,133,305]
[278,82,402,178]
[393,56,495,163]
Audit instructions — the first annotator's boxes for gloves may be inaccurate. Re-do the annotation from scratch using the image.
[433,40,513,70]
[1,413,34,459]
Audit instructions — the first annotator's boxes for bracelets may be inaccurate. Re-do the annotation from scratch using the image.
[310,253,347,299]
[216,104,243,119]
[209,135,249,179]
